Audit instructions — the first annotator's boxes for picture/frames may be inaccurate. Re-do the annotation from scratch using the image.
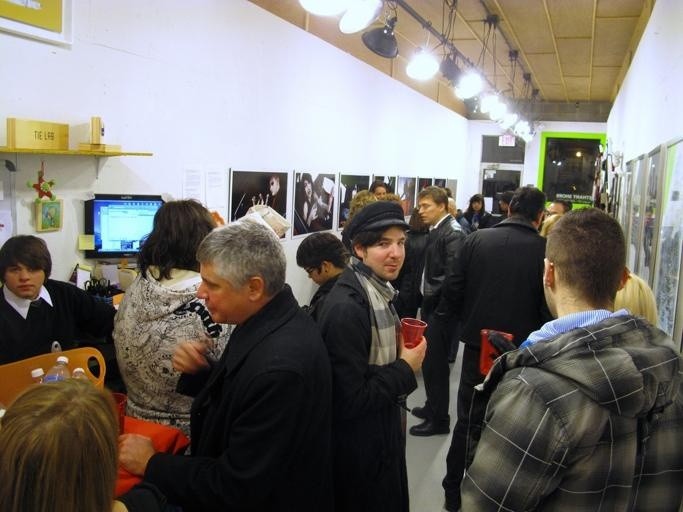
[228,167,456,244]
[619,137,681,356]
[1,0,74,47]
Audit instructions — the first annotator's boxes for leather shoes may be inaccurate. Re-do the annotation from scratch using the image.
[410,406,450,435]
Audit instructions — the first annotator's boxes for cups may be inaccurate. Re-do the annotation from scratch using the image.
[105,391,128,436]
[400,317,428,349]
[479,327,514,376]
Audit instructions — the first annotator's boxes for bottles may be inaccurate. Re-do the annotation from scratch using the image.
[30,355,86,385]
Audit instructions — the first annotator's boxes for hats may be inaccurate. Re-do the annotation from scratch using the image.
[342,200,412,250]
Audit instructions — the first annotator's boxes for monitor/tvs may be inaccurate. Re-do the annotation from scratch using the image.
[85,194,167,258]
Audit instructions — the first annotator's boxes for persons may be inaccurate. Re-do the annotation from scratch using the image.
[341,189,378,246]
[267,173,286,219]
[302,173,334,231]
[111,200,239,456]
[113,218,333,511]
[294,232,352,317]
[368,180,683,512]
[316,201,429,510]
[0,234,125,394]
[0,377,126,511]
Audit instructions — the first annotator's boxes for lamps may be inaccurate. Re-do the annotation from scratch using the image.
[297,0,543,144]
